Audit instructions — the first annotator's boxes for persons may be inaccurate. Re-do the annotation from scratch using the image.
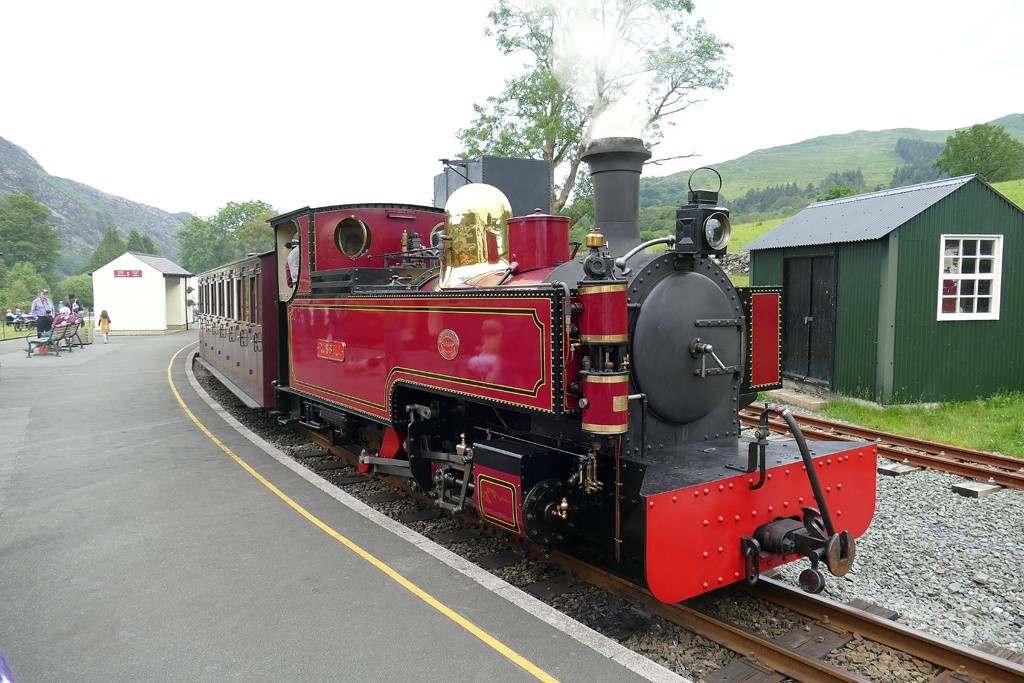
[344,235,361,255]
[99,311,111,343]
[285,247,300,293]
[6,289,84,347]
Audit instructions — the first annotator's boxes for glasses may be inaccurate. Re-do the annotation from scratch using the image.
[44,292,48,295]
[60,313,65,315]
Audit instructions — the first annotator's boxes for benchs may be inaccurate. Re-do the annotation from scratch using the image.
[27,323,84,358]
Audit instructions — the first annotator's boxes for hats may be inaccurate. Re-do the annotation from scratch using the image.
[69,293,75,298]
[41,289,48,292]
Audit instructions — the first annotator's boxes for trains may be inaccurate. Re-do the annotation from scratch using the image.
[197,135,877,606]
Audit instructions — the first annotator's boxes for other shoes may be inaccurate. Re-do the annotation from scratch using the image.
[24,347,34,353]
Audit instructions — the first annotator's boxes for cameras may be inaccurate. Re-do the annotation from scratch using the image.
[46,310,52,314]
